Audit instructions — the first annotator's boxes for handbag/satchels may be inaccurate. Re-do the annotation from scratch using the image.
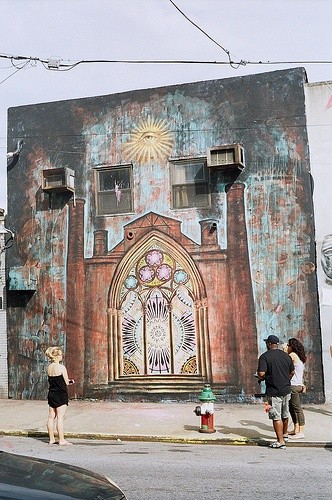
[300,385,307,395]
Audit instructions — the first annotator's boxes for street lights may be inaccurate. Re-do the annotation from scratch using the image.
[0,208,18,312]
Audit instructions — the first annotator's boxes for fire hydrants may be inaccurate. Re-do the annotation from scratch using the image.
[193,383,217,434]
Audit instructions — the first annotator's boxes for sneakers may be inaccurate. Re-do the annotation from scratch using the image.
[288,432,305,439]
[288,429,295,435]
[269,442,286,450]
[283,433,288,438]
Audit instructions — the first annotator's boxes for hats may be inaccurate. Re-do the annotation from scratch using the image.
[263,335,280,344]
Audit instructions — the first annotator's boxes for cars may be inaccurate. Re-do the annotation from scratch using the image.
[0,451,128,500]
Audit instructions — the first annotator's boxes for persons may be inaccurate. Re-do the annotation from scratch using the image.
[255,335,295,449]
[45,347,75,446]
[282,338,306,439]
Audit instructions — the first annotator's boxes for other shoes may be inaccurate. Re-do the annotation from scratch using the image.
[48,440,73,447]
